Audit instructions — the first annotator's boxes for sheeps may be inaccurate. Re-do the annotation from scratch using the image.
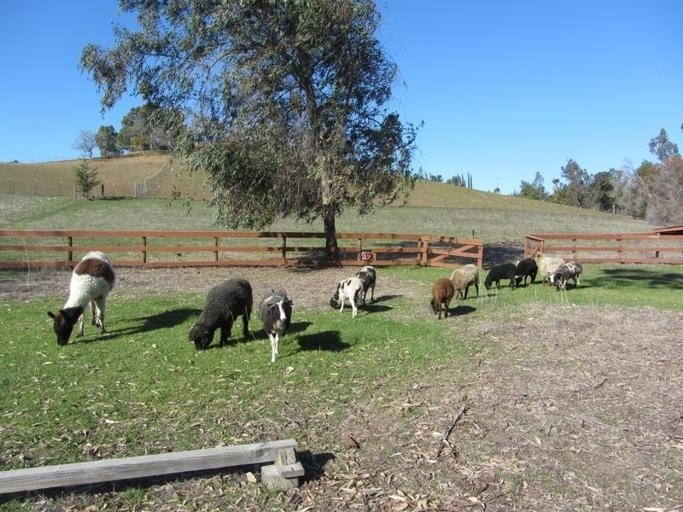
[450,264,479,300]
[330,277,363,317]
[258,288,294,364]
[188,277,253,351]
[354,266,375,304]
[485,251,582,290]
[46,251,116,345]
[429,278,454,320]
